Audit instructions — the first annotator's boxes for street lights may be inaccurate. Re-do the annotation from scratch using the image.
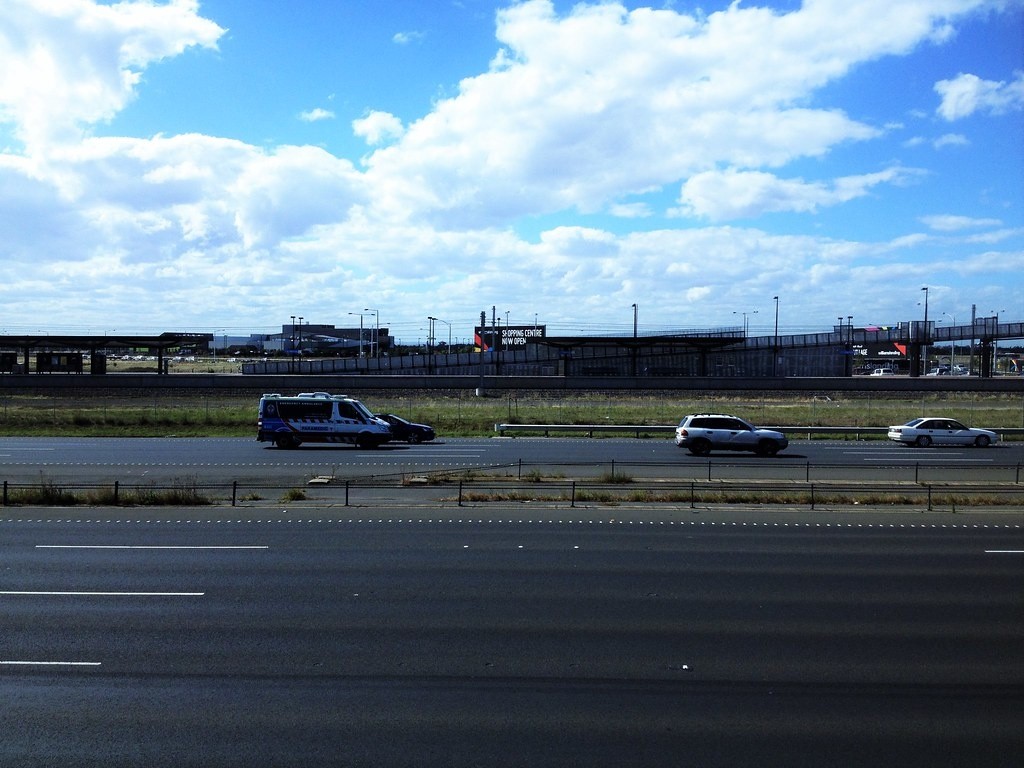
[347,286,1023,354]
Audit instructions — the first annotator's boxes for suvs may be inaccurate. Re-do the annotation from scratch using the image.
[676,414,790,455]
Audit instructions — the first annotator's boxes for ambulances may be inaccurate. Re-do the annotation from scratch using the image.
[257,390,393,451]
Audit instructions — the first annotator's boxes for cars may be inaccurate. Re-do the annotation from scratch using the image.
[375,415,438,445]
[887,418,1001,447]
[926,368,950,376]
[870,368,894,376]
[954,363,967,371]
